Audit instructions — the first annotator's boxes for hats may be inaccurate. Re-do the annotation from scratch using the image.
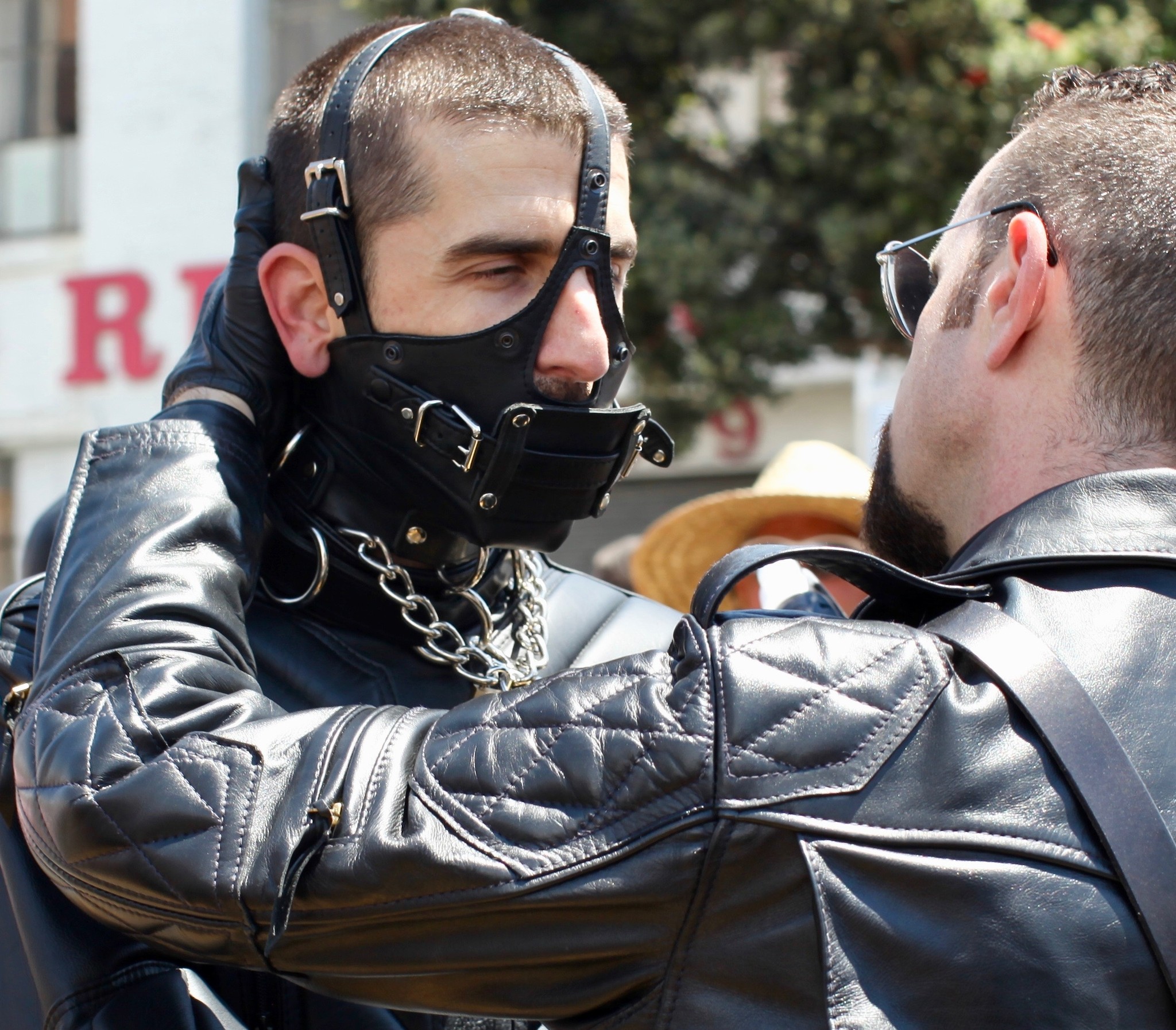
[628,436,876,612]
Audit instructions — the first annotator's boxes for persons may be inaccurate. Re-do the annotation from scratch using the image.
[0,7,679,1030]
[11,60,1176,1030]
[592,438,884,628]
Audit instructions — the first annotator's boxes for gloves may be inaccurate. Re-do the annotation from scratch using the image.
[156,155,288,434]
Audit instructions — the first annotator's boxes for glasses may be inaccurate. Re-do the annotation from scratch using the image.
[875,201,1059,347]
